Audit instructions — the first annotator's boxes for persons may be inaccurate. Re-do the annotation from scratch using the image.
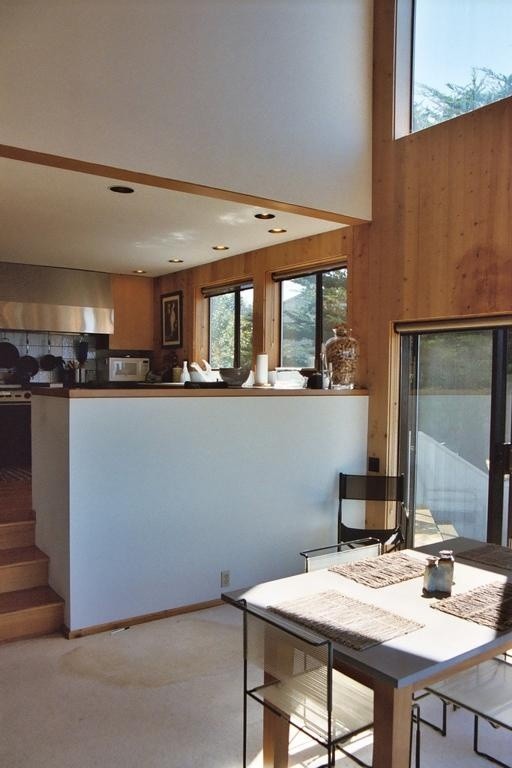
[168,303,176,337]
[163,301,170,337]
[173,321,177,337]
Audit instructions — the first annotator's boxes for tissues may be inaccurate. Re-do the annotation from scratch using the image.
[189,359,221,382]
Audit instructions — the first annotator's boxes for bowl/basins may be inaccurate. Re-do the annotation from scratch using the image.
[219,367,250,389]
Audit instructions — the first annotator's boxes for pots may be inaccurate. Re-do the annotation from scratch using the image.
[39,338,58,371]
[18,339,39,377]
[0,338,20,369]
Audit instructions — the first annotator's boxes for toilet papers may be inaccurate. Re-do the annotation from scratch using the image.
[255,354,269,385]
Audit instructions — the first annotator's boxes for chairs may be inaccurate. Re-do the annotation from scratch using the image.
[338,471,410,558]
[232,595,396,764]
[296,540,390,588]
[420,648,512,768]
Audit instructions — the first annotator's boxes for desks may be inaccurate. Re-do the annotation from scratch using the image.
[219,536,512,766]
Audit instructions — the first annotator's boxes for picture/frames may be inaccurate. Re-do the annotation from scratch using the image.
[160,291,183,348]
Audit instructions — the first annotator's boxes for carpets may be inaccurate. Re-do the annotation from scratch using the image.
[0,464,32,482]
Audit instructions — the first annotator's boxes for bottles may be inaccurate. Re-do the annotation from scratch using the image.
[422,556,438,598]
[436,548,455,598]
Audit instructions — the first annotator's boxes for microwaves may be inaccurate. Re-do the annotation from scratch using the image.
[104,356,151,383]
